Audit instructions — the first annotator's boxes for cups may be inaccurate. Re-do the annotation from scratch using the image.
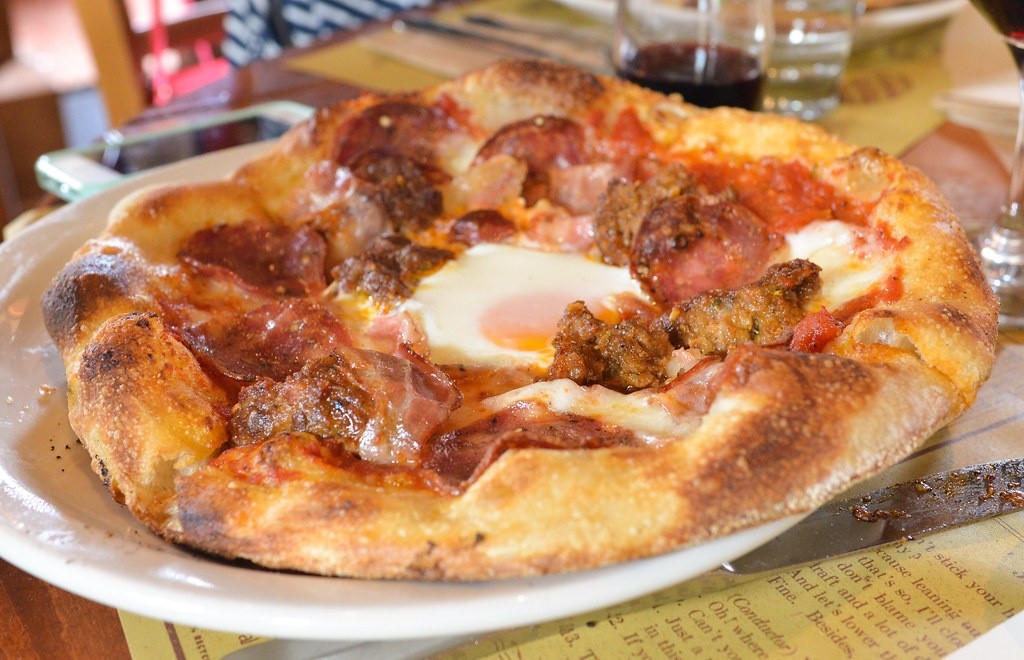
[753,1,862,119]
[610,0,768,110]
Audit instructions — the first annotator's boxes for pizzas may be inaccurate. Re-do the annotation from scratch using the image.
[39,56,1002,581]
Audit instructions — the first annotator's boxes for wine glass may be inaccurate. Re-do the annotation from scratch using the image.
[962,1,1024,335]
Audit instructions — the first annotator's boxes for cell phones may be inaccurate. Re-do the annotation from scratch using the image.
[35,98,317,199]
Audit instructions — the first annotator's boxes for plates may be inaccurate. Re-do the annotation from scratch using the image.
[2,127,839,641]
[623,0,973,55]
